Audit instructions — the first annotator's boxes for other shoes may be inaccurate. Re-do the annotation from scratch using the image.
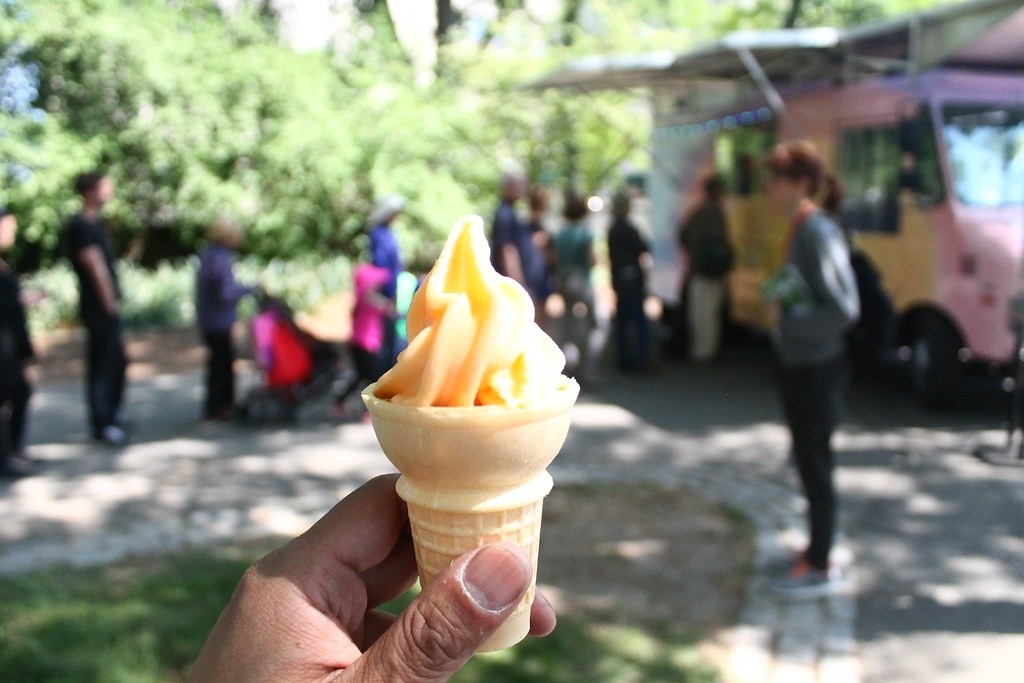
[99,411,137,447]
[1,455,47,475]
[211,403,249,421]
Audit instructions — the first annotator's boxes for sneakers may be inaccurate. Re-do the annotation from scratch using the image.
[766,551,844,602]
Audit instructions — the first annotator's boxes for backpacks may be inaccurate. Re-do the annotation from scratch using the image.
[844,250,897,381]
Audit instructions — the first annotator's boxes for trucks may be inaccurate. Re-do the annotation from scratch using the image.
[469,0,1024,421]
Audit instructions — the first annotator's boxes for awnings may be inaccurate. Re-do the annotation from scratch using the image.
[518,28,840,176]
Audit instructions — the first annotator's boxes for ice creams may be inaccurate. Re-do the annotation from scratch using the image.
[362,211,580,653]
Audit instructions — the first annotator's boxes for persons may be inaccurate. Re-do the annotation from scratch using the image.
[63,172,131,445]
[194,172,734,420]
[0,211,44,478]
[193,473,557,683]
[883,149,925,231]
[764,144,864,593]
